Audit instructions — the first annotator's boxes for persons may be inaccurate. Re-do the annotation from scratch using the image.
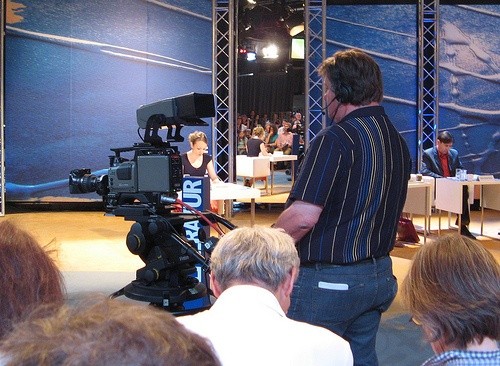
[171,224,354,365]
[0,219,66,340]
[421,130,475,240]
[270,47,411,365]
[177,132,219,182]
[236,108,306,186]
[1,296,222,365]
[405,231,500,366]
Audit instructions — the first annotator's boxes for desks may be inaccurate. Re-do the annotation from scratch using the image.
[240,152,298,196]
[435,173,500,237]
[404,173,433,248]
[210,181,262,231]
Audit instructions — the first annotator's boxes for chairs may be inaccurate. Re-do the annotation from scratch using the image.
[409,168,477,237]
[235,155,271,195]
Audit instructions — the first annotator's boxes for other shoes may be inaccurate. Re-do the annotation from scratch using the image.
[461,225,477,239]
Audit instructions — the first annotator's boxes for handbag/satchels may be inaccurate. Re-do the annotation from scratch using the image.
[397,213,420,243]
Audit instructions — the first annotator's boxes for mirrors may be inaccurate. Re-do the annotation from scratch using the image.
[234,0,306,204]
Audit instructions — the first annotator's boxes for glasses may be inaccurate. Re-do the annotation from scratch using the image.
[409,314,424,326]
